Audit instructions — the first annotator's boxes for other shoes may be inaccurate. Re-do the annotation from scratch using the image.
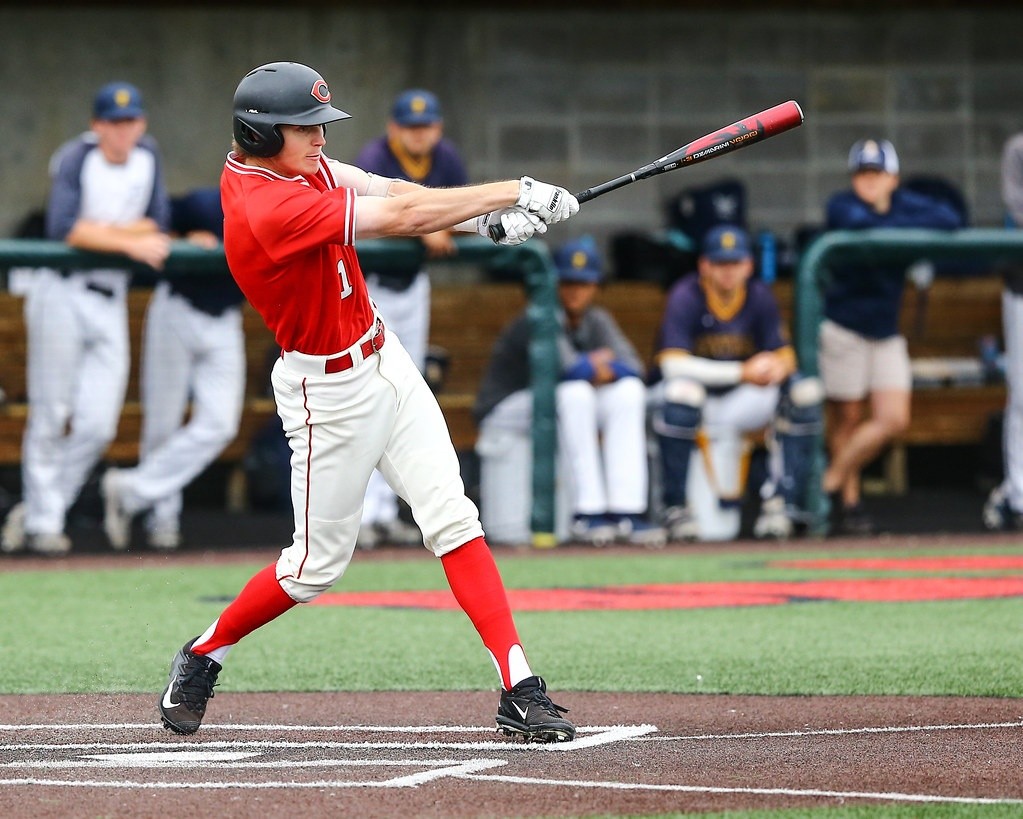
[652,505,697,543]
[753,495,791,540]
[92,466,131,550]
[1,500,72,558]
[147,531,180,550]
[570,513,664,544]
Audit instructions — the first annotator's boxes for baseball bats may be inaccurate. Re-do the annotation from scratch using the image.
[487,98,806,245]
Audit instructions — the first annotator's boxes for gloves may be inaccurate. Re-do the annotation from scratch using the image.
[477,175,580,246]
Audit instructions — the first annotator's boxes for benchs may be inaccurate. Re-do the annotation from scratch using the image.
[0,278,1013,467]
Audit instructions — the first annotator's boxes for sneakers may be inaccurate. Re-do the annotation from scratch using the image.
[157,635,222,736]
[495,676,575,742]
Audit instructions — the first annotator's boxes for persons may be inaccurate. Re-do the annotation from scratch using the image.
[471,236,669,546]
[808,139,961,534]
[354,91,468,547]
[646,225,793,542]
[982,134,1023,529]
[2,62,577,743]
[3,83,171,556]
[100,189,246,550]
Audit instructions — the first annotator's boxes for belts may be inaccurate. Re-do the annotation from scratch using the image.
[281,301,385,374]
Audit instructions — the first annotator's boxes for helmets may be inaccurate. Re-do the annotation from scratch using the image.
[233,62,353,158]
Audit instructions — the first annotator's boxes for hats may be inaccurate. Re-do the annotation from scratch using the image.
[552,236,602,283]
[846,134,900,175]
[391,91,440,127]
[703,225,750,261]
[95,82,144,120]
